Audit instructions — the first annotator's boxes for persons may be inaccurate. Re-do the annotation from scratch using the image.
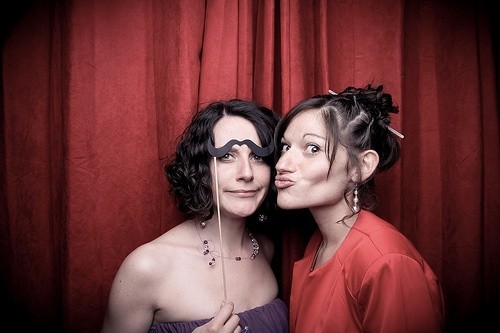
[99,99,290,333]
[275,83,445,333]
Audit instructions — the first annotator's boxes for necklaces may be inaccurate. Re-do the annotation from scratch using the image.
[310,237,337,271]
[190,219,261,266]
